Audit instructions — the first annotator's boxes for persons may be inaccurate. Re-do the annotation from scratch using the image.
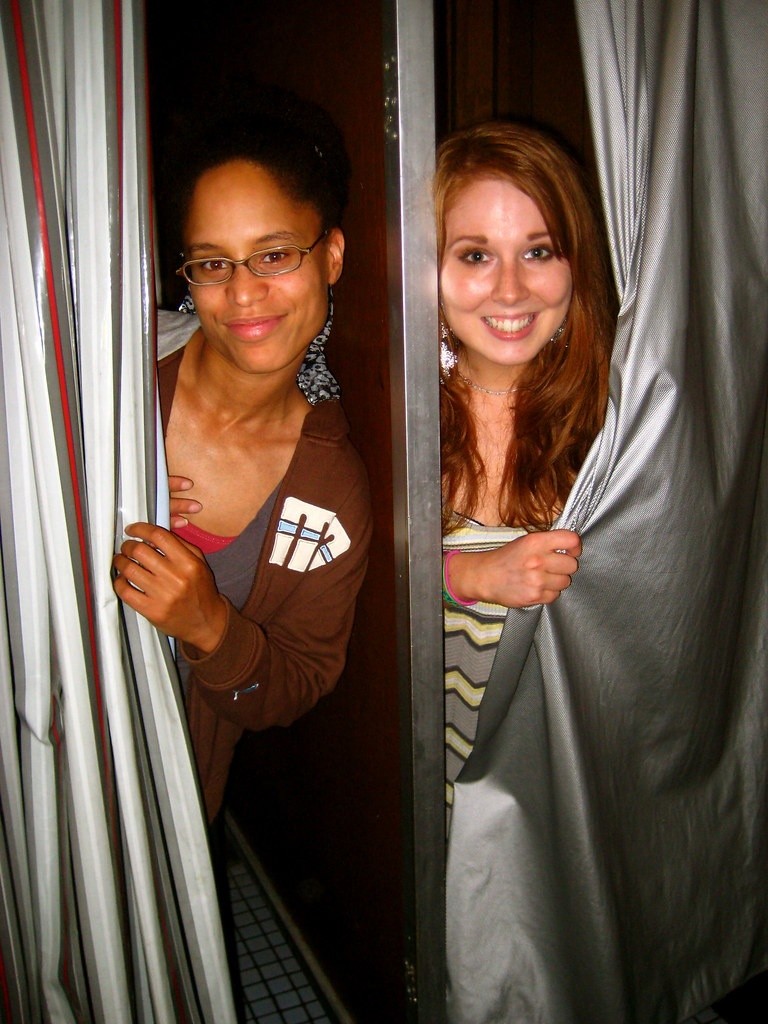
[113,95,374,827]
[352,119,617,842]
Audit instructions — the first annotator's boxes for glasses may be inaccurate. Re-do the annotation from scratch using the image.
[175,228,331,286]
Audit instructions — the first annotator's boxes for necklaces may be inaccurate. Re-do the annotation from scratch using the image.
[457,371,518,396]
